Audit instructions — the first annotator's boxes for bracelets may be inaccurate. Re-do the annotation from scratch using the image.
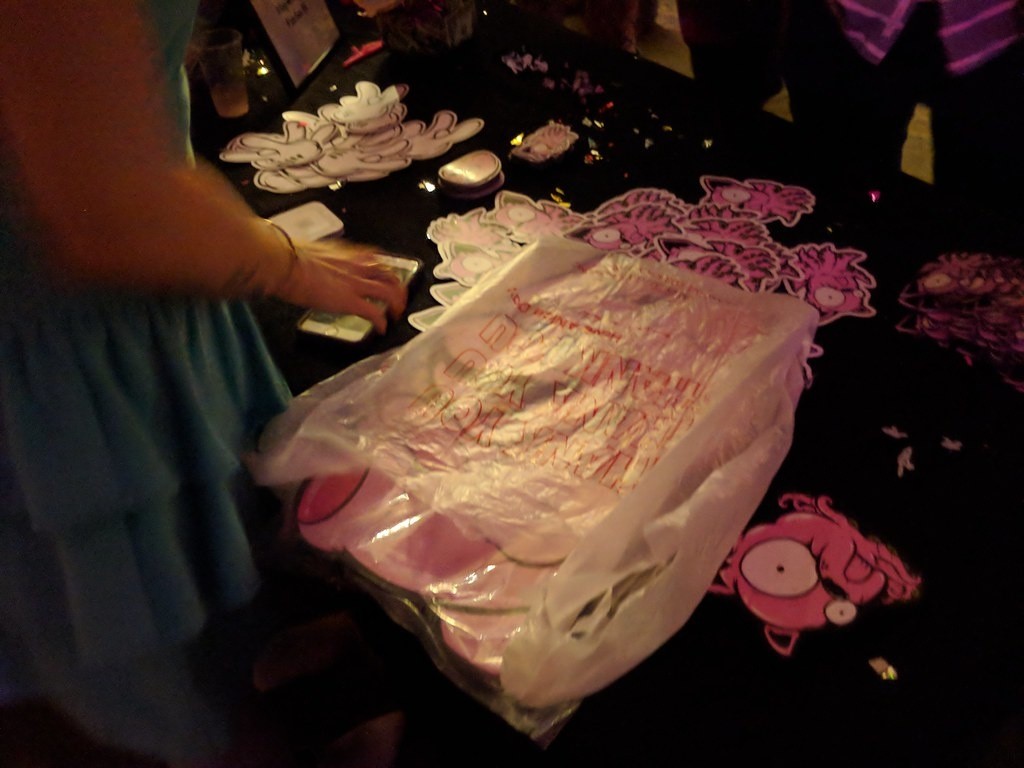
[263,219,302,309]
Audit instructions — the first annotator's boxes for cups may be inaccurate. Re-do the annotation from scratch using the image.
[196,29,249,116]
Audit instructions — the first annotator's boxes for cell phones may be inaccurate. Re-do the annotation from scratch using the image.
[295,250,424,344]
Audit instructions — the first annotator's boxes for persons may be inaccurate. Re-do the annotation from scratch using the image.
[502,0,1024,218]
[0,0,407,767]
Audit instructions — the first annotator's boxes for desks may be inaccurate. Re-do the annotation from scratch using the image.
[190,0,1024,768]
